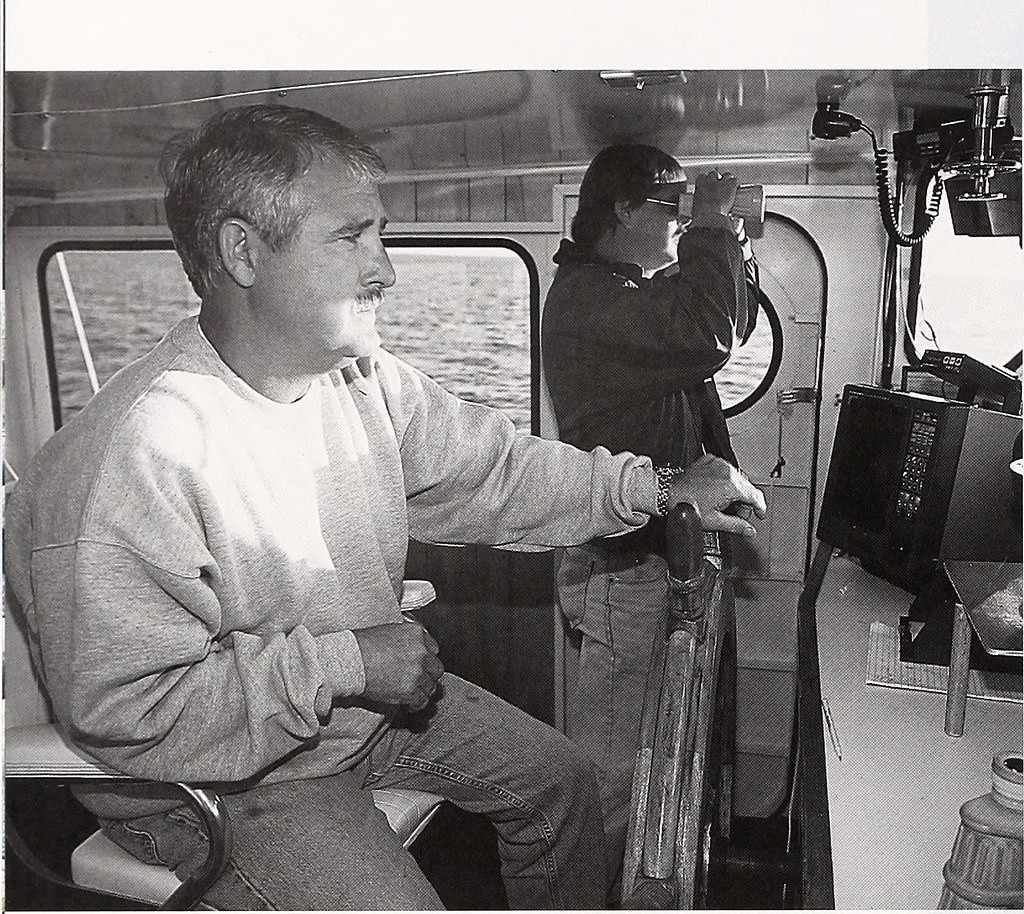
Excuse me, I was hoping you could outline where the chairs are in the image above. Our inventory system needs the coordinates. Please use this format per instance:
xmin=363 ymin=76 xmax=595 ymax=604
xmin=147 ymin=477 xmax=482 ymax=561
xmin=3 ymin=479 xmax=445 ymax=902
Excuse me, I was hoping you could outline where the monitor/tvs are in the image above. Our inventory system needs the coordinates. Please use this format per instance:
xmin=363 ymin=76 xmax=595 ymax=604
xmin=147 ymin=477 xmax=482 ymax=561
xmin=828 ymin=396 xmax=910 ymax=541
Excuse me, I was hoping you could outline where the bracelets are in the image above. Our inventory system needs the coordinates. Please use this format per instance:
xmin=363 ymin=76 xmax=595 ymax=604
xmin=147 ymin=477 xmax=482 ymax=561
xmin=656 ymin=467 xmax=673 ymax=516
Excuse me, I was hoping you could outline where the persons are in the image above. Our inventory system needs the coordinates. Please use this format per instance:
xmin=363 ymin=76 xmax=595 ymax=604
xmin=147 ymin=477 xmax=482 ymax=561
xmin=3 ymin=105 xmax=768 ymax=910
xmin=538 ymin=146 xmax=758 ymax=898
